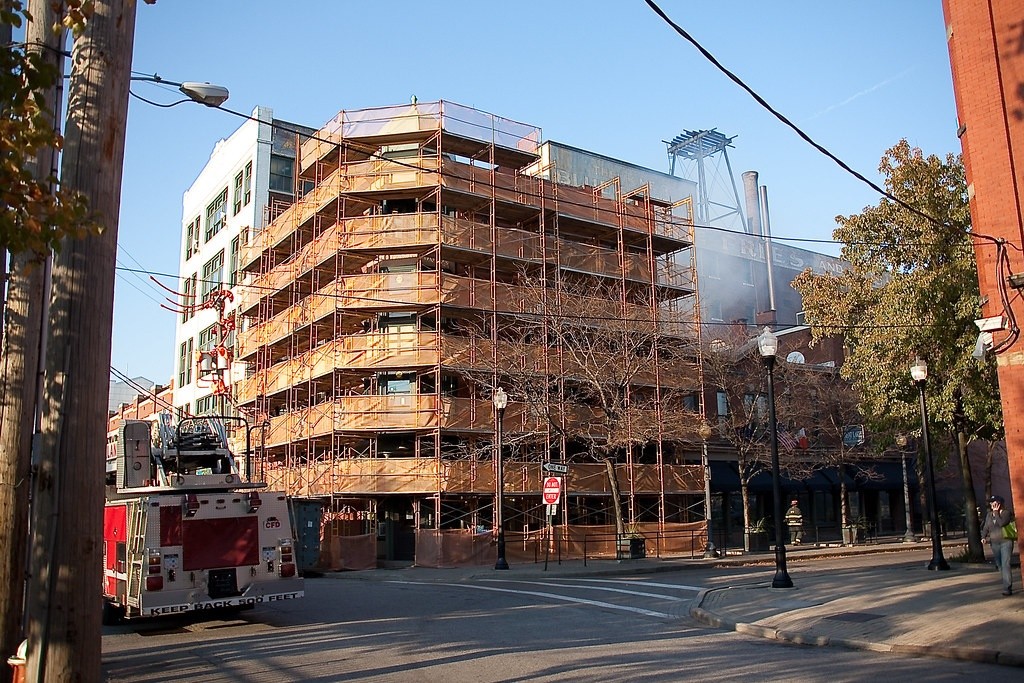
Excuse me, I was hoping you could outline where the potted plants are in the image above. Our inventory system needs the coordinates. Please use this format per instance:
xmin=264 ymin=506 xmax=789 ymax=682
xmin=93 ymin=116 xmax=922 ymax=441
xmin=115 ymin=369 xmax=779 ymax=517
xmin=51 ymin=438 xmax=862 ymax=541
xmin=844 ymin=513 xmax=874 ymax=545
xmin=750 ymin=517 xmax=769 ymax=552
xmin=618 ymin=518 xmax=645 ymax=559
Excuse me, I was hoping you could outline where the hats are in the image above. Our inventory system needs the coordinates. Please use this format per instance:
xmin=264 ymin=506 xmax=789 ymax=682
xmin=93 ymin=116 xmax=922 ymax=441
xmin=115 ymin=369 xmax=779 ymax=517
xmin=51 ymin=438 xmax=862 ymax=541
xmin=790 ymin=500 xmax=798 ymax=505
xmin=985 ymin=495 xmax=1005 ymax=504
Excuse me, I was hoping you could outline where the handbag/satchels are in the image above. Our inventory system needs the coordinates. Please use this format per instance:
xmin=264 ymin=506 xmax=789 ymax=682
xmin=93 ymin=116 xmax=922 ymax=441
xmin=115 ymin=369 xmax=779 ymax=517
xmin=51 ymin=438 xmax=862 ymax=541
xmin=1000 ymin=508 xmax=1017 ymax=539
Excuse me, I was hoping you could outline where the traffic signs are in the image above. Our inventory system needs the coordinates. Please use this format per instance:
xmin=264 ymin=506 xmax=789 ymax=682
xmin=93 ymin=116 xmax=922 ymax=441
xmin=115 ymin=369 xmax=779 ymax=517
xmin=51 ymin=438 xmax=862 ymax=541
xmin=540 ymin=460 xmax=568 ymax=475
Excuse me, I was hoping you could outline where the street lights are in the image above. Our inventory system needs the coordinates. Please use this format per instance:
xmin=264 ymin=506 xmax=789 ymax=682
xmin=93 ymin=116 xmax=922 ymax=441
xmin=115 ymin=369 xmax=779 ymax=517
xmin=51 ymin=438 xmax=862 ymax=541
xmin=492 ymin=386 xmax=509 ymax=572
xmin=755 ymin=326 xmax=795 ymax=589
xmin=893 ymin=431 xmax=917 ymax=543
xmin=906 ymin=354 xmax=951 ymax=571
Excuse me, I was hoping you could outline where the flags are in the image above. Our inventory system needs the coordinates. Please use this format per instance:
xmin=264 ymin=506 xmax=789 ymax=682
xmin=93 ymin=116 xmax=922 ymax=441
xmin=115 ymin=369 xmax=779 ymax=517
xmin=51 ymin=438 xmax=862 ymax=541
xmin=767 ymin=420 xmax=798 ymax=452
xmin=794 ymin=428 xmax=808 ymax=449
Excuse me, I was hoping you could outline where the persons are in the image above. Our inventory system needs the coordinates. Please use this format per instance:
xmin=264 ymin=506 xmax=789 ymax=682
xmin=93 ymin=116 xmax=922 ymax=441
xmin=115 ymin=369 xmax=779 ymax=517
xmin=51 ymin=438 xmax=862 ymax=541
xmin=785 ymin=500 xmax=803 ymax=546
xmin=981 ymin=495 xmax=1014 ymax=595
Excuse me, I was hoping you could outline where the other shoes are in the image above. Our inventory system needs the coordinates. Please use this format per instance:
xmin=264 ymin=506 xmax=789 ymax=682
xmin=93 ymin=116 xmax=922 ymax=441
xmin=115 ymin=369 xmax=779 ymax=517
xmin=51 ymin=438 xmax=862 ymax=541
xmin=1001 ymin=591 xmax=1013 ymax=596
xmin=791 ymin=544 xmax=797 ymax=546
xmin=795 ymin=541 xmax=803 ymax=545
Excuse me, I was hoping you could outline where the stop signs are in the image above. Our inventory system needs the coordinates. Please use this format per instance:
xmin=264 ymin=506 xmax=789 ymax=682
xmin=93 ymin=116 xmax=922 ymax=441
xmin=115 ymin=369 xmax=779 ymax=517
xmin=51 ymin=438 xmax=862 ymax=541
xmin=542 ymin=476 xmax=562 ymax=506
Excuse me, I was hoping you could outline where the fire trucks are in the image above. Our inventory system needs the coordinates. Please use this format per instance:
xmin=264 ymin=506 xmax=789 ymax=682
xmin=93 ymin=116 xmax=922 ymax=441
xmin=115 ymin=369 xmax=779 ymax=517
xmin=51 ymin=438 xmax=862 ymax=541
xmin=100 ymin=415 xmax=306 ymax=627
